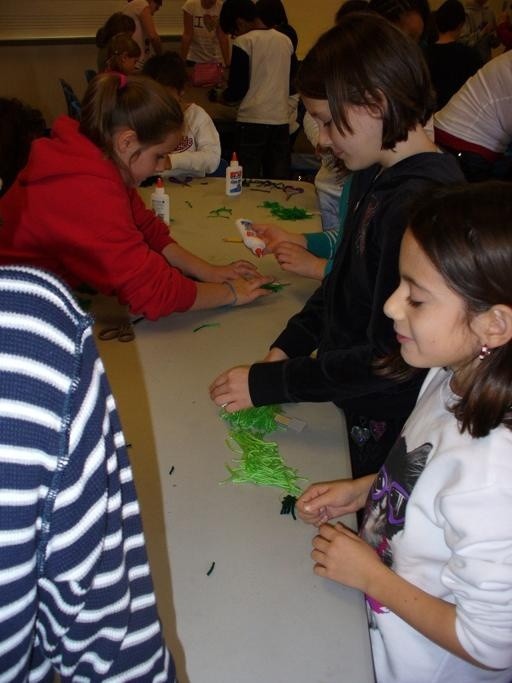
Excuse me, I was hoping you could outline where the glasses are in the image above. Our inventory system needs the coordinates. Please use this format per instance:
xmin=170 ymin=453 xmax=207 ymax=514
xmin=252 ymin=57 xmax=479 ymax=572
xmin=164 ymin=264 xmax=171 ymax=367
xmin=369 ymin=465 xmax=410 ymax=524
xmin=231 ymin=30 xmax=235 ymax=39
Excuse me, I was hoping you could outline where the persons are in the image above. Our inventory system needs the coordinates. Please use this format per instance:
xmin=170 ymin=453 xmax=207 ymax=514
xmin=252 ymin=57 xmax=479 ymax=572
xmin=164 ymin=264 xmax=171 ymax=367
xmin=296 ymin=195 xmax=511 ymax=677
xmin=120 ymin=0 xmax=159 ymax=51
xmin=252 ymin=170 xmax=352 ymax=281
xmin=181 ymin=1 xmax=232 ymax=78
xmin=98 ymin=36 xmax=141 ymax=67
xmin=216 ymin=2 xmax=291 ymax=173
xmin=423 ymin=4 xmax=486 ymax=104
xmin=133 ymin=56 xmax=222 ymax=182
xmin=259 ymin=4 xmax=301 ymax=169
xmin=424 ymin=47 xmax=509 ymax=179
xmin=303 ymin=1 xmax=424 ymax=230
xmin=0 ymin=268 xmax=176 ymax=683
xmin=0 ymin=70 xmax=278 ymax=319
xmin=209 ymin=20 xmax=473 ymax=480
xmin=94 ymin=13 xmax=134 ymax=67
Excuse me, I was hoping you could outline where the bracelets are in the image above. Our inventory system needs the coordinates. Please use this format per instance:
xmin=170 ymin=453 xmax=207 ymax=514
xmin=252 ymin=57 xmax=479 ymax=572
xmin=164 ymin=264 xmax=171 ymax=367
xmin=223 ymin=280 xmax=236 ymax=311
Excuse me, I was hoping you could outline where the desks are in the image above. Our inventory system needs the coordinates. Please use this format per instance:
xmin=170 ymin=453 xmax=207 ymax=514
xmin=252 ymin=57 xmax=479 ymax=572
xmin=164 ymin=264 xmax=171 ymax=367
xmin=87 ymin=176 xmax=376 ymax=682
xmin=160 ymin=84 xmax=322 ymax=153
